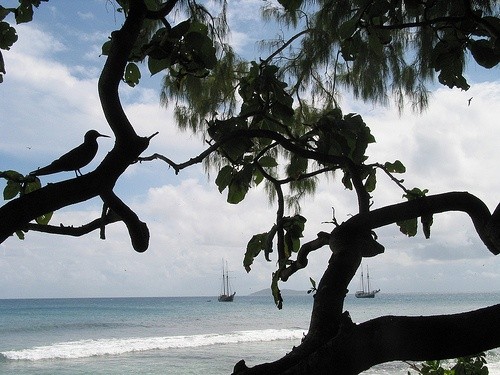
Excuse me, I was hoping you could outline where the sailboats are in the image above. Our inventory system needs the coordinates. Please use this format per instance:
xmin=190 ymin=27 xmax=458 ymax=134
xmin=354 ymin=263 xmax=381 ymax=299
xmin=218 ymin=261 xmax=237 ymax=303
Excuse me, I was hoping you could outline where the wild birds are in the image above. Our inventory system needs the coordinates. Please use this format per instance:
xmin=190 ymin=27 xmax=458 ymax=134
xmin=28 ymin=129 xmax=111 ymax=180
